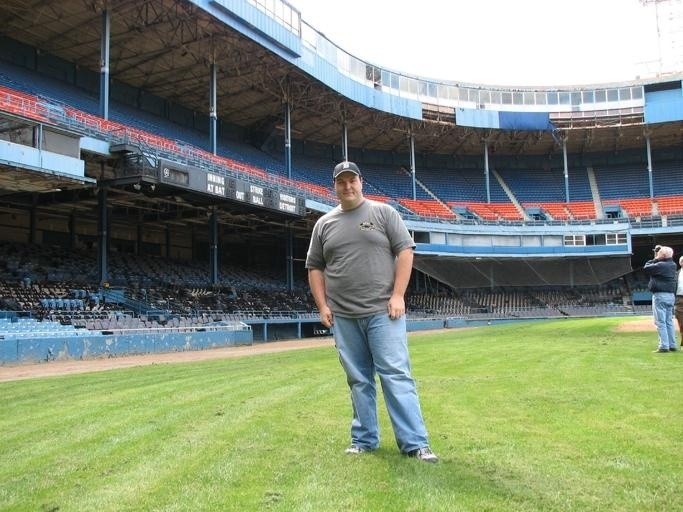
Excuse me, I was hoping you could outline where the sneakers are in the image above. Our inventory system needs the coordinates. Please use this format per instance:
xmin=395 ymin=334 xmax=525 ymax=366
xmin=345 ymin=445 xmax=366 ymax=454
xmin=653 ymin=348 xmax=668 ymax=353
xmin=408 ymin=446 xmax=439 ymax=462
xmin=668 ymin=348 xmax=677 ymax=351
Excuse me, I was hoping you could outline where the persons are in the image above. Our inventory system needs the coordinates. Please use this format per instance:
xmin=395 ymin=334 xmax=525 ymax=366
xmin=305 ymin=161 xmax=439 ymax=464
xmin=644 ymin=243 xmax=679 ymax=352
xmin=674 ymin=255 xmax=683 ymax=350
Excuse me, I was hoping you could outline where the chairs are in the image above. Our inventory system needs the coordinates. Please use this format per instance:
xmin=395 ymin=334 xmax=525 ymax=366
xmin=1 ymin=64 xmax=103 ymax=140
xmin=405 ymin=281 xmax=652 ymax=318
xmin=414 ymin=167 xmax=683 ymax=221
xmin=99 ymin=251 xmax=321 ymax=334
xmin=1 ymin=242 xmax=99 ymax=338
xmin=103 ymin=99 xmax=213 ymax=171
xmin=213 ymin=136 xmax=414 ymax=216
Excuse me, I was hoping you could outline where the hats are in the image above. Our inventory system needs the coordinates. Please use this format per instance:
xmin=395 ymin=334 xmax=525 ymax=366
xmin=332 ymin=161 xmax=361 ymax=179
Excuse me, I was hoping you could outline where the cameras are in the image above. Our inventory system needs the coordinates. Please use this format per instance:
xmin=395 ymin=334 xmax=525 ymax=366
xmin=652 ymin=247 xmax=661 ymax=258
